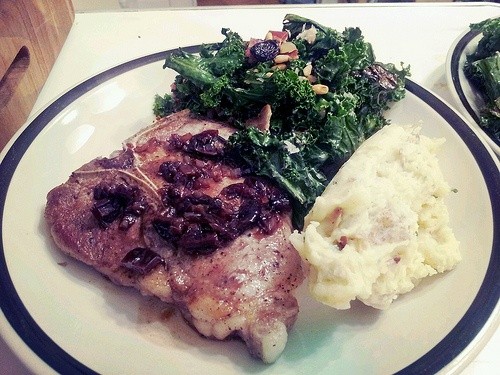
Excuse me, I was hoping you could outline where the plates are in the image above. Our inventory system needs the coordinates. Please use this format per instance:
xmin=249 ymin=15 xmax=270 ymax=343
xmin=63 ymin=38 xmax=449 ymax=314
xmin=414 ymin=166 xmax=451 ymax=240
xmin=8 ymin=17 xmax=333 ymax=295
xmin=448 ymin=15 xmax=500 ymax=153
xmin=0 ymin=43 xmax=499 ymax=375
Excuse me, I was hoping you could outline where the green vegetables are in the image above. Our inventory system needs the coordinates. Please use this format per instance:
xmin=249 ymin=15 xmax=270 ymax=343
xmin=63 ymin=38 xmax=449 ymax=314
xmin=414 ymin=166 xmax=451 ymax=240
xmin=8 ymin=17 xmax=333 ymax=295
xmin=465 ymin=16 xmax=500 ymax=143
xmin=153 ymin=14 xmax=412 ymax=234
